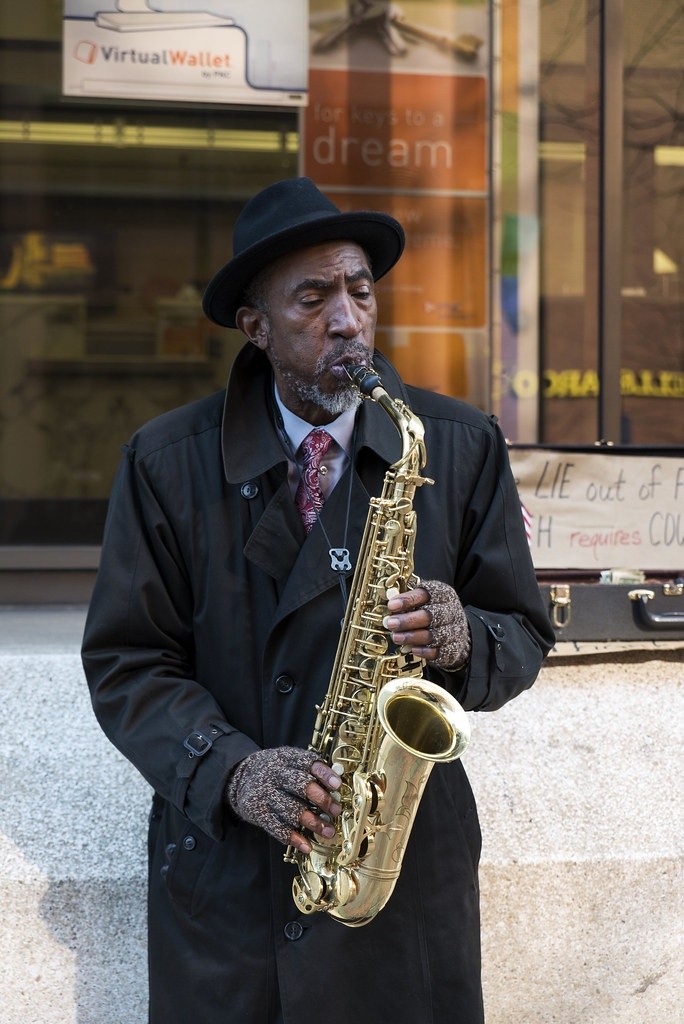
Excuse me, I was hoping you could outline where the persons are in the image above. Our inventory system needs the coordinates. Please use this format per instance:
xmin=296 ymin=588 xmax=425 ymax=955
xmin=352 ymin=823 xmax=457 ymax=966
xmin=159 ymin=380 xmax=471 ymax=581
xmin=80 ymin=175 xmax=557 ymax=1024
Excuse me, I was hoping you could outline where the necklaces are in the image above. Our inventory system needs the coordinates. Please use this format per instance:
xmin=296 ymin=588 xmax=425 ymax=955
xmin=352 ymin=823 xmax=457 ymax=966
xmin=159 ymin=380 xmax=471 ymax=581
xmin=271 ymin=395 xmax=360 ymax=612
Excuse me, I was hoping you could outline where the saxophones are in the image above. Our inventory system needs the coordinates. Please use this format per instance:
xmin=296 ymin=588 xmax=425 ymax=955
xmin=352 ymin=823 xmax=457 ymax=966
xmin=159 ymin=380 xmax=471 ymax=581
xmin=284 ymin=357 xmax=474 ymax=928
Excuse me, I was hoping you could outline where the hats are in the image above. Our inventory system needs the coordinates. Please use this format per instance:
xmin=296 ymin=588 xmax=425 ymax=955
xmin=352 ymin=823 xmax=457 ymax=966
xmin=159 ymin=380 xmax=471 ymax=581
xmin=202 ymin=177 xmax=407 ymax=330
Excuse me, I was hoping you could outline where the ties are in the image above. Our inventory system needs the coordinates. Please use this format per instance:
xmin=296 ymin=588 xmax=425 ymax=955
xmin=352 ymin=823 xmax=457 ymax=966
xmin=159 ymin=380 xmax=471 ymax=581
xmin=297 ymin=429 xmax=333 ymax=534
